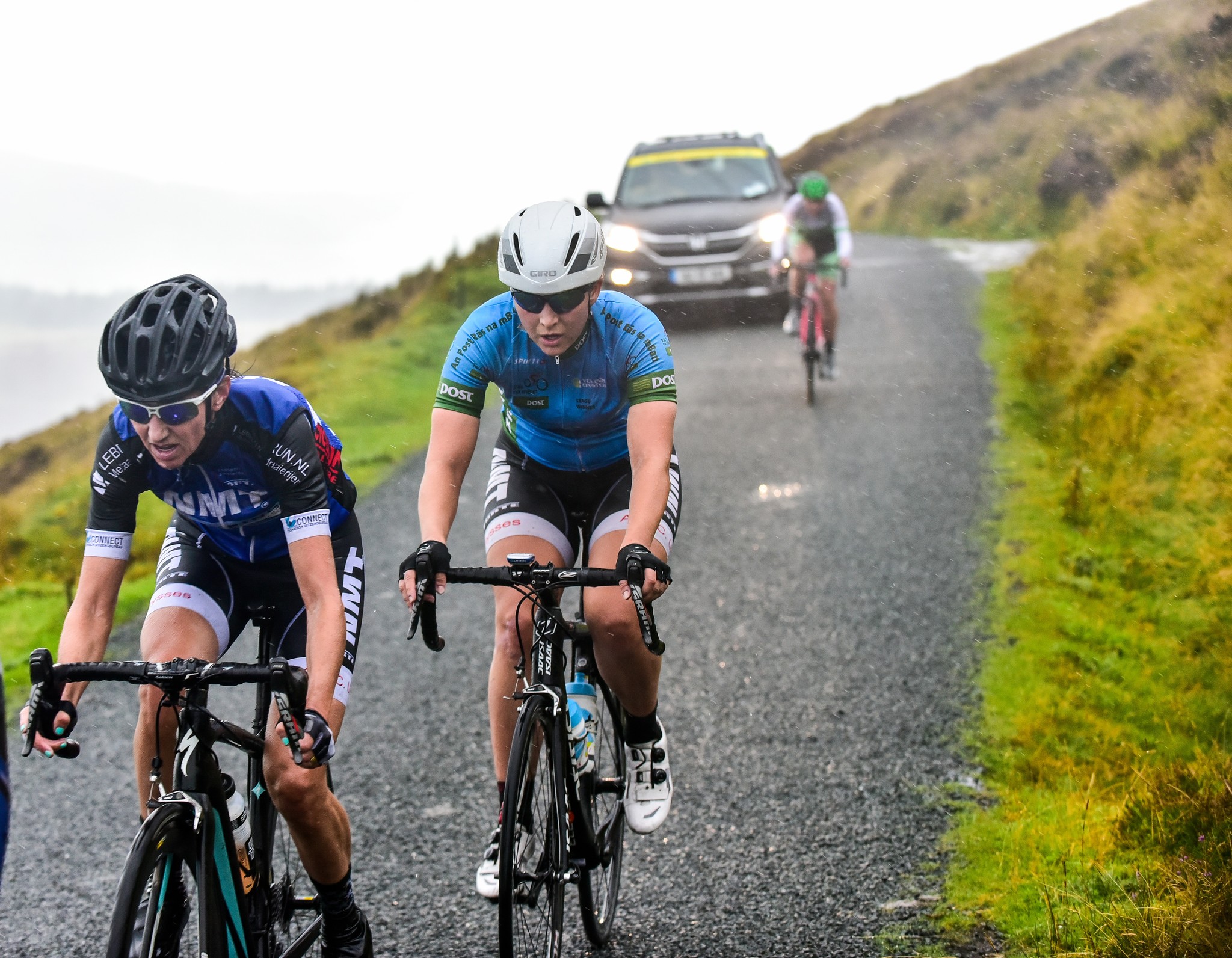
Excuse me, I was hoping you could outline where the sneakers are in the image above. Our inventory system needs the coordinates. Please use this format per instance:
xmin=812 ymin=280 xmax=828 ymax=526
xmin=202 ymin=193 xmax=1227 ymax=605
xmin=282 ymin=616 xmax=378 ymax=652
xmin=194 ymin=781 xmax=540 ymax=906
xmin=622 ymin=715 xmax=674 ymax=834
xmin=475 ymin=822 xmax=535 ymax=899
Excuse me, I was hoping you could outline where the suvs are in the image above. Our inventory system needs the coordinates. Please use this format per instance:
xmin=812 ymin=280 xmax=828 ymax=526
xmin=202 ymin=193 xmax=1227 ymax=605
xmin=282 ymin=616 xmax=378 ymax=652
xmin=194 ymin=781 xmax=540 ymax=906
xmin=583 ymin=130 xmax=800 ymax=322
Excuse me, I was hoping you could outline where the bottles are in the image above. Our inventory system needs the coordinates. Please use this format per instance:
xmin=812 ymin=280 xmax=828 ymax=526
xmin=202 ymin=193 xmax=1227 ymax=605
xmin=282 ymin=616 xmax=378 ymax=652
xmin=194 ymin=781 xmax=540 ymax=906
xmin=568 ymin=698 xmax=589 ymax=775
xmin=221 ymin=773 xmax=257 ymax=894
xmin=565 ymin=673 xmax=597 ymax=773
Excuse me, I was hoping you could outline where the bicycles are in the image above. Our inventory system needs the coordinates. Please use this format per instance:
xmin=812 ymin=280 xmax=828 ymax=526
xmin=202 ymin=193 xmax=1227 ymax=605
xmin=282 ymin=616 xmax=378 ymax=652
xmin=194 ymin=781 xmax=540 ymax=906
xmin=20 ymin=581 xmax=321 ymax=958
xmin=774 ymin=248 xmax=849 ymax=406
xmin=404 ymin=509 xmax=667 ymax=958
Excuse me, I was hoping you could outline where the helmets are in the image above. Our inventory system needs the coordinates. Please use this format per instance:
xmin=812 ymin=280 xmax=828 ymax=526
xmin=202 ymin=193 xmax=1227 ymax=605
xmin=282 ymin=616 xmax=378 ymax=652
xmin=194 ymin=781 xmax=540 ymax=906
xmin=798 ymin=175 xmax=828 ymax=201
xmin=98 ymin=274 xmax=238 ymax=406
xmin=498 ymin=201 xmax=607 ymax=296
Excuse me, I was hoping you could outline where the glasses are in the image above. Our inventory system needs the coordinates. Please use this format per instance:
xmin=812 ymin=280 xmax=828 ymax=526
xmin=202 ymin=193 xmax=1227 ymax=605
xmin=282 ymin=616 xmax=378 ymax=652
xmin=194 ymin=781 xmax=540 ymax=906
xmin=512 ymin=288 xmax=587 ymax=314
xmin=118 ymin=384 xmax=216 ymax=425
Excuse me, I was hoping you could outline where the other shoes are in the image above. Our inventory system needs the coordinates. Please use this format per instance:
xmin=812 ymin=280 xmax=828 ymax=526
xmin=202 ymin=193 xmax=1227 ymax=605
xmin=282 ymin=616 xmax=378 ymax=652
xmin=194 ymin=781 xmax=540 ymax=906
xmin=320 ymin=906 xmax=370 ymax=958
xmin=782 ymin=313 xmax=799 ymax=335
xmin=824 ymin=362 xmax=838 ymax=379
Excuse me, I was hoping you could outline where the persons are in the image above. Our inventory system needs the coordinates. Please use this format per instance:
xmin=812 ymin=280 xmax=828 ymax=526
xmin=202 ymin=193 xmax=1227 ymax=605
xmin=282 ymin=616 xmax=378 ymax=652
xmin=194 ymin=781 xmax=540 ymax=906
xmin=771 ymin=173 xmax=851 ymax=378
xmin=20 ymin=273 xmax=373 ymax=958
xmin=397 ymin=200 xmax=682 ymax=898
